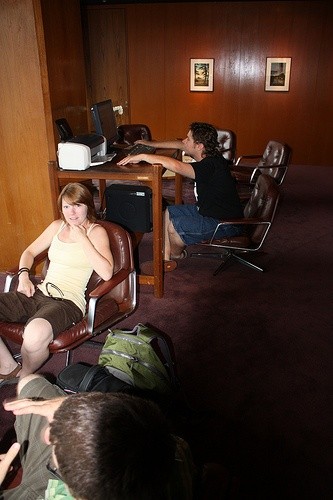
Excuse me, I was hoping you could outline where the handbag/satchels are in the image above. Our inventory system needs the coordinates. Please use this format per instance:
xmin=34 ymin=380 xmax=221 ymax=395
xmin=56 ymin=362 xmax=137 ymax=394
xmin=8 ymin=268 xmax=35 ymax=293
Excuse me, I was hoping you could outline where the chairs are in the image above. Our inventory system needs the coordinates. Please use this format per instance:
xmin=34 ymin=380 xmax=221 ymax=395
xmin=0 ymin=219 xmax=139 ymax=368
xmin=190 ymin=174 xmax=281 ymax=277
xmin=217 ymin=130 xmax=236 ymax=163
xmin=228 ymin=140 xmax=293 ymax=203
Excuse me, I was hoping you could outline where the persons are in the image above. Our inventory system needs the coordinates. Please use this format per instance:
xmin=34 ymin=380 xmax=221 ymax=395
xmin=116 ymin=122 xmax=244 ymax=274
xmin=0 ymin=183 xmax=113 ymax=388
xmin=0 ymin=374 xmax=193 ymax=500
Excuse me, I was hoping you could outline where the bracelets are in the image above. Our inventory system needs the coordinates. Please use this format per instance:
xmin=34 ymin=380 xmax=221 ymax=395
xmin=17 ymin=268 xmax=30 ymax=276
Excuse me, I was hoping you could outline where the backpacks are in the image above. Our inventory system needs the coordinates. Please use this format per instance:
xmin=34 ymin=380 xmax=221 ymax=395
xmin=98 ymin=323 xmax=171 ymax=391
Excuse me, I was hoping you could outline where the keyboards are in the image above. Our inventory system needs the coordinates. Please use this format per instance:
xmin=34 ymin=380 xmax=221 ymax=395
xmin=126 ymin=143 xmax=156 ymax=164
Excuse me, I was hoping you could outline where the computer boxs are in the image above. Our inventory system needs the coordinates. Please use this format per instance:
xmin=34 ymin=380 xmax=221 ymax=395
xmin=104 ymin=183 xmax=154 ymax=233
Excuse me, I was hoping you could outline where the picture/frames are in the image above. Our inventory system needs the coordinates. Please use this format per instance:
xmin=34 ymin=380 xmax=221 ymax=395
xmin=189 ymin=58 xmax=215 ymax=92
xmin=264 ymin=57 xmax=292 ymax=92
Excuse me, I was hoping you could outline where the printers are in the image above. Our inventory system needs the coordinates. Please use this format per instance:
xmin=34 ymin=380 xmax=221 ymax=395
xmin=55 ymin=118 xmax=117 ymax=171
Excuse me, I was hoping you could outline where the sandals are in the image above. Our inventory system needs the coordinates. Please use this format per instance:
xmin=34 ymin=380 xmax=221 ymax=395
xmin=0 ymin=362 xmax=22 ymax=387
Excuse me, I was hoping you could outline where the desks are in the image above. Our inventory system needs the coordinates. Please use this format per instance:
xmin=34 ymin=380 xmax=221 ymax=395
xmin=48 ymin=148 xmax=182 ymax=298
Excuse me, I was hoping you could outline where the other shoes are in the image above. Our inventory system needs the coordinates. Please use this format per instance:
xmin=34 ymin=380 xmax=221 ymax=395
xmin=139 ymin=249 xmax=187 ymax=274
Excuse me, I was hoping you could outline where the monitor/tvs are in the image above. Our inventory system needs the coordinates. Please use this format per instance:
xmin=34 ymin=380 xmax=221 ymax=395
xmin=92 ymin=99 xmax=119 ymax=154
